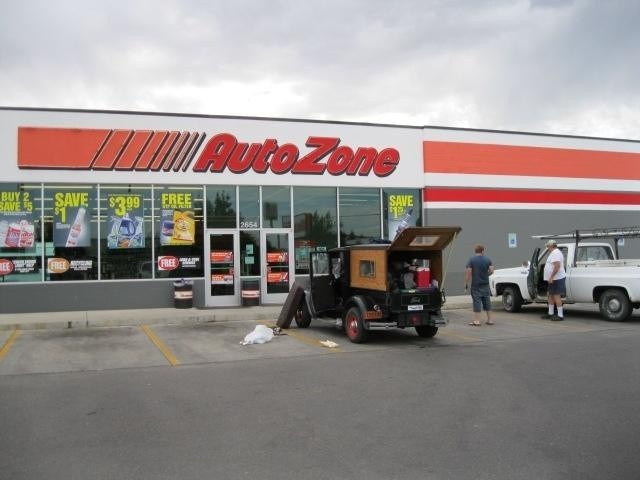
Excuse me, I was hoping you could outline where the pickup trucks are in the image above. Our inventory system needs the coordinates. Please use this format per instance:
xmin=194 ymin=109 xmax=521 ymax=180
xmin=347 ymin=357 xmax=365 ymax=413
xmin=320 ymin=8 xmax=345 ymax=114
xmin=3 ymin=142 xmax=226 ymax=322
xmin=486 ymin=228 xmax=640 ymax=323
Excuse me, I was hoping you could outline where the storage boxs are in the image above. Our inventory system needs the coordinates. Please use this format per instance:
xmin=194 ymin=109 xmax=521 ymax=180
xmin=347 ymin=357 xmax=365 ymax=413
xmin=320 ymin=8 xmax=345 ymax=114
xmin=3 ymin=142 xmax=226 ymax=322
xmin=407 ymin=265 xmax=431 ymax=288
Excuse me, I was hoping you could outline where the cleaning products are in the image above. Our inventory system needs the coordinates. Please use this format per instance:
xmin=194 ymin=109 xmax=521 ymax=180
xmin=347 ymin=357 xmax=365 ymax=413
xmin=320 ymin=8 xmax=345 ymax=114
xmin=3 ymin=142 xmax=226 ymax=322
xmin=128 ymin=216 xmax=144 ymax=248
xmin=106 ymin=216 xmax=122 ymax=248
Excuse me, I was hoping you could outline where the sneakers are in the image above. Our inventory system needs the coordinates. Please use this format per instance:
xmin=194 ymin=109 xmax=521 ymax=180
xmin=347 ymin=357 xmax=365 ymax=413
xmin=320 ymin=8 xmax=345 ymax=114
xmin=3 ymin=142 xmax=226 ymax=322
xmin=541 ymin=314 xmax=565 ymax=321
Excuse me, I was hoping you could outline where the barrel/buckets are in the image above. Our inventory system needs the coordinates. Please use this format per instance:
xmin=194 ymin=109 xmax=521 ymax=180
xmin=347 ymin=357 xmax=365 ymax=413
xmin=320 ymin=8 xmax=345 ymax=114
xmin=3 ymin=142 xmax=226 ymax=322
xmin=170 ymin=210 xmax=195 ymax=245
xmin=117 ymin=213 xmax=136 ymax=248
xmin=159 ymin=207 xmax=176 ymax=246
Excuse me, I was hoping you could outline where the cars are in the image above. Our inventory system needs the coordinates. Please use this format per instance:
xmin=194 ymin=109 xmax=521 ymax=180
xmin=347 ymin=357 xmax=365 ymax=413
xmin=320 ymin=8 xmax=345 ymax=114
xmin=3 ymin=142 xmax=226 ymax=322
xmin=140 ymin=260 xmax=168 ymax=279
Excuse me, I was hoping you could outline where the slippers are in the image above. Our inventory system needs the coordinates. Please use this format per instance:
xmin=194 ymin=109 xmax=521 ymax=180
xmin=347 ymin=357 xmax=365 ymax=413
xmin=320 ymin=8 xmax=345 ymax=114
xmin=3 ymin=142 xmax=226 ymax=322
xmin=468 ymin=321 xmax=480 ymax=326
xmin=485 ymin=320 xmax=494 ymax=325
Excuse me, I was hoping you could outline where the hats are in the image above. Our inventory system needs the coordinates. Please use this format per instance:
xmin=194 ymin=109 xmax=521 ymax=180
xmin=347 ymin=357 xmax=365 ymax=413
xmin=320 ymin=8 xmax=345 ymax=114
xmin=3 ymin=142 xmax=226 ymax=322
xmin=544 ymin=239 xmax=557 ymax=246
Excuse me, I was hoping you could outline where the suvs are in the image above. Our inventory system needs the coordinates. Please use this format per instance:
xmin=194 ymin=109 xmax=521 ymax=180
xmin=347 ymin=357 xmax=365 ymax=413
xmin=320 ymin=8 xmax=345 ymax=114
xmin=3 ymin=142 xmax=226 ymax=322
xmin=277 ymin=223 xmax=463 ymax=342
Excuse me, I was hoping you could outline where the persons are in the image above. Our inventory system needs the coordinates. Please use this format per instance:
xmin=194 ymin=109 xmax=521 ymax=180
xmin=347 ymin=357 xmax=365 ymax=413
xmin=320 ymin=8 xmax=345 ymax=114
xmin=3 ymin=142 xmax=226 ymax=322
xmin=463 ymin=244 xmax=495 ymax=327
xmin=539 ymin=238 xmax=567 ymax=322
